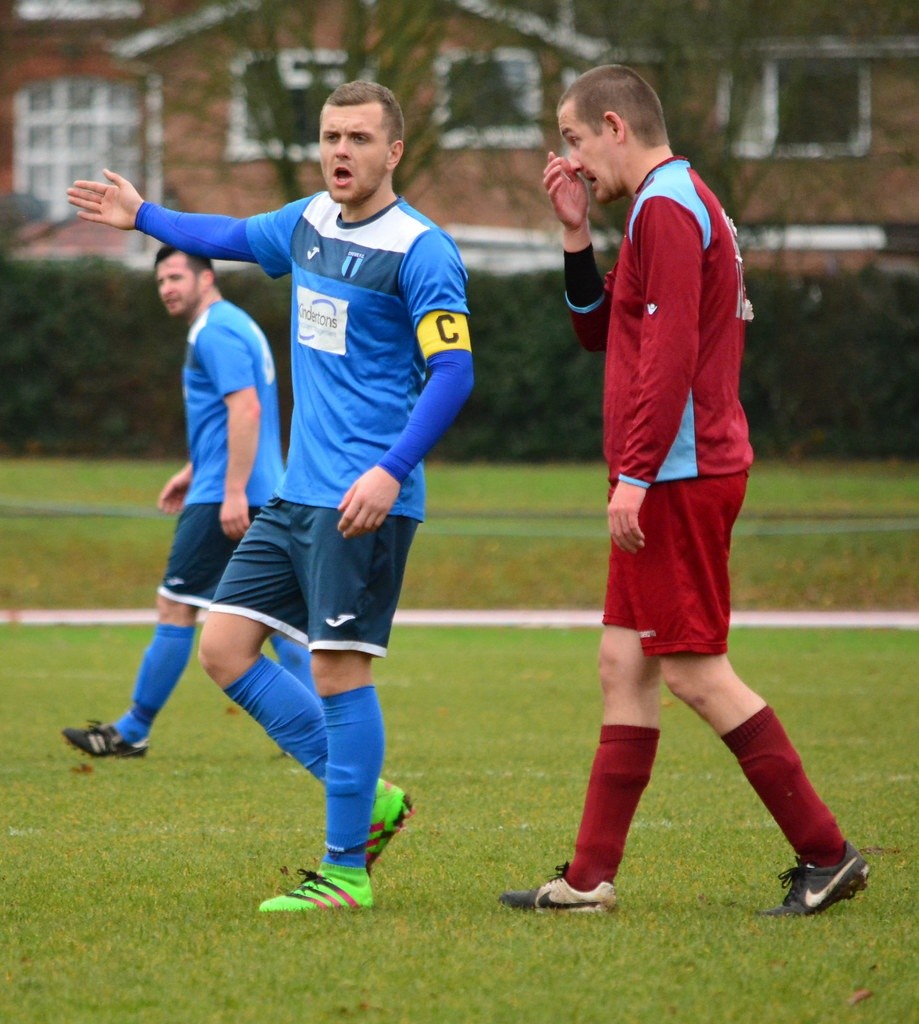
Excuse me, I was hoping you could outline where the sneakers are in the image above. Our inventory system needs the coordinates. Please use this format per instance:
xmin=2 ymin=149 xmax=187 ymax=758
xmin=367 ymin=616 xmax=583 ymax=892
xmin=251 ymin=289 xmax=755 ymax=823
xmin=364 ymin=777 xmax=417 ymax=870
xmin=754 ymin=841 xmax=871 ymax=916
xmin=60 ymin=719 xmax=148 ymax=757
xmin=260 ymin=861 xmax=373 ymax=911
xmin=497 ymin=862 xmax=615 ymax=912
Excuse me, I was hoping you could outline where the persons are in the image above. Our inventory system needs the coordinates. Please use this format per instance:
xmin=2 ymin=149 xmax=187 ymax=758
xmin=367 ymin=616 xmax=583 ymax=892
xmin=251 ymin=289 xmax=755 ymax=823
xmin=61 ymin=247 xmax=313 ymax=757
xmin=67 ymin=81 xmax=472 ymax=911
xmin=496 ymin=64 xmax=871 ymax=918
